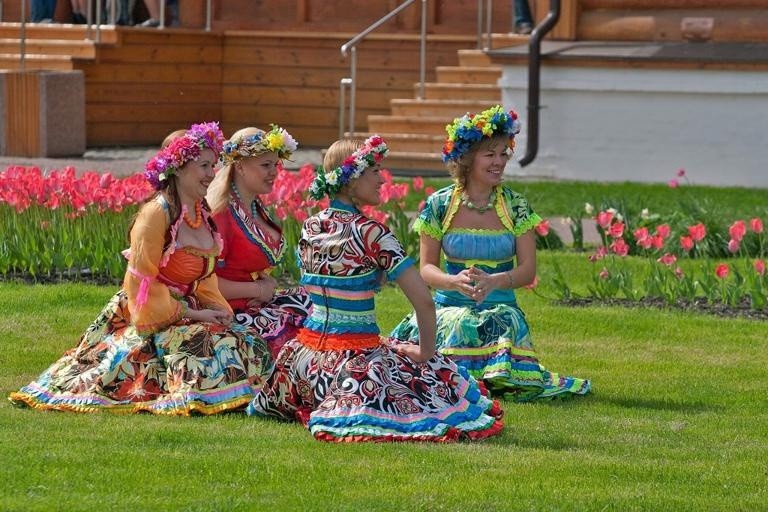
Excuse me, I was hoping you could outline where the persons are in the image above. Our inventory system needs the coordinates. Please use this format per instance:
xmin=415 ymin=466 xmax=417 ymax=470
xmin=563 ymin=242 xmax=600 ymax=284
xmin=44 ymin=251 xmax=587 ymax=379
xmin=30 ymin=0 xmax=180 ymax=27
xmin=513 ymin=0 xmax=536 ymax=34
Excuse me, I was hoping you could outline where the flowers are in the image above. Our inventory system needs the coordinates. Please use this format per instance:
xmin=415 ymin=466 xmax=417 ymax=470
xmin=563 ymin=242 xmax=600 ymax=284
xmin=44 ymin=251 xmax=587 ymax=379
xmin=144 ymin=118 xmax=224 ymax=191
xmin=442 ymin=104 xmax=521 ymax=163
xmin=308 ymin=134 xmax=389 ymax=202
xmin=223 ymin=123 xmax=299 ymax=163
